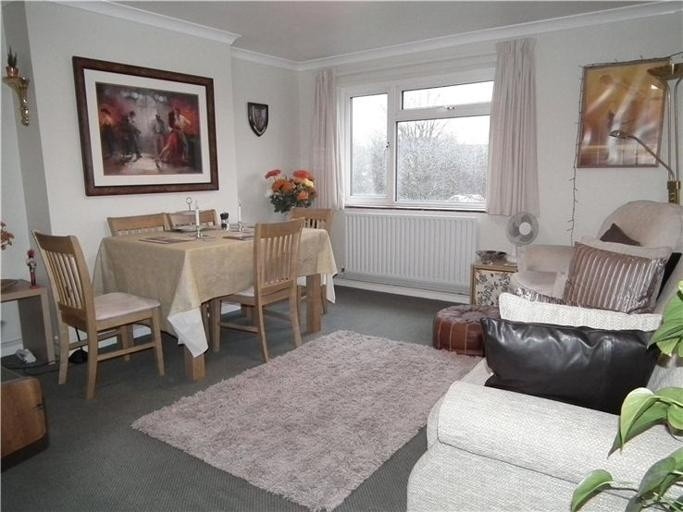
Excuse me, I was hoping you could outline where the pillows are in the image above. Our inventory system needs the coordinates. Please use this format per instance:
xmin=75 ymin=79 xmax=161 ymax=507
xmin=484 ymin=321 xmax=662 ymax=411
xmin=551 ymin=222 xmax=681 ymax=314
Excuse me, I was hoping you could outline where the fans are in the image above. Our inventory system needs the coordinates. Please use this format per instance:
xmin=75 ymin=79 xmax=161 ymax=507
xmin=509 ymin=211 xmax=540 ymax=264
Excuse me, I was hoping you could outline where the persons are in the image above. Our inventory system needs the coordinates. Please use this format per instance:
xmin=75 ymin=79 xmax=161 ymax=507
xmin=253 ymin=107 xmax=264 ymax=130
xmin=99 ymin=105 xmax=193 ymax=169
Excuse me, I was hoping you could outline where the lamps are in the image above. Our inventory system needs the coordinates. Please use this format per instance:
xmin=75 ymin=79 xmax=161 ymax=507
xmin=609 ymin=63 xmax=683 ymax=206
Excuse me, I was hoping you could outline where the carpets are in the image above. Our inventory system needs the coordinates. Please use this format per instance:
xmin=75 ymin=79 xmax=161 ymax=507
xmin=133 ymin=329 xmax=483 ymax=510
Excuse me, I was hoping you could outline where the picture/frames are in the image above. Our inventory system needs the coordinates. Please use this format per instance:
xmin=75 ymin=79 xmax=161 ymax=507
xmin=248 ymin=101 xmax=271 ymax=137
xmin=68 ymin=56 xmax=220 ymax=195
xmin=575 ymin=56 xmax=668 ymax=169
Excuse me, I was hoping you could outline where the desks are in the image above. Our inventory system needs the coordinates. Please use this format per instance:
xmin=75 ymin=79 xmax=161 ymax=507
xmin=1 ymin=277 xmax=56 ymax=369
xmin=470 ymin=262 xmax=518 ymax=309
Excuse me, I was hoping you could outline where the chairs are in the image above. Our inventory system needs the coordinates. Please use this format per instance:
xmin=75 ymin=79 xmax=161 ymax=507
xmin=405 ymin=291 xmax=683 ymax=511
xmin=511 ymin=199 xmax=683 ymax=310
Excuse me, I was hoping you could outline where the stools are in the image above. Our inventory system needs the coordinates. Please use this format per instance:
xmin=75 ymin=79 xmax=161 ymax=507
xmin=431 ymin=304 xmax=500 ymax=357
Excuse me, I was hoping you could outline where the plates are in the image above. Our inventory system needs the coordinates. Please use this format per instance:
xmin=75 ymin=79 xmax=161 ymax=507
xmin=172 ymin=222 xmax=256 ymax=240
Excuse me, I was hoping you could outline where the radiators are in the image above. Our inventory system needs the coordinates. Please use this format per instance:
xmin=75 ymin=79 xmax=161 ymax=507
xmin=344 ymin=211 xmax=478 ymax=299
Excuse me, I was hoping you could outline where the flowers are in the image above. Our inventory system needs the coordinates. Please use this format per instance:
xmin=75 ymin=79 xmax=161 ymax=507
xmin=265 ymin=169 xmax=314 ymax=213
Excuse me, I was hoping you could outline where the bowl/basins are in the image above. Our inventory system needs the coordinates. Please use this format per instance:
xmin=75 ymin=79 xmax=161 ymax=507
xmin=475 ymin=249 xmax=508 ymax=266
